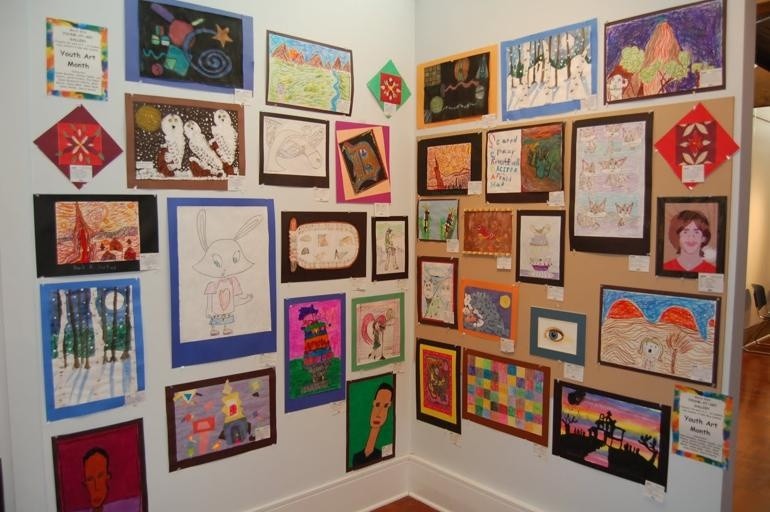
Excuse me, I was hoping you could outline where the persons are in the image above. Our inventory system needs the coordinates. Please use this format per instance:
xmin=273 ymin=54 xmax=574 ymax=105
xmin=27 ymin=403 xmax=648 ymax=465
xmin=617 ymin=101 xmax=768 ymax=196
xmin=662 ymin=208 xmax=718 ymax=273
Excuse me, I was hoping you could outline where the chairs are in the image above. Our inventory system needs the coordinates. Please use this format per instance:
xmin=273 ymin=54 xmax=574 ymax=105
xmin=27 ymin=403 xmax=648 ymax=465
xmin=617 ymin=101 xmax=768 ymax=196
xmin=743 ymin=284 xmax=770 ymax=356
